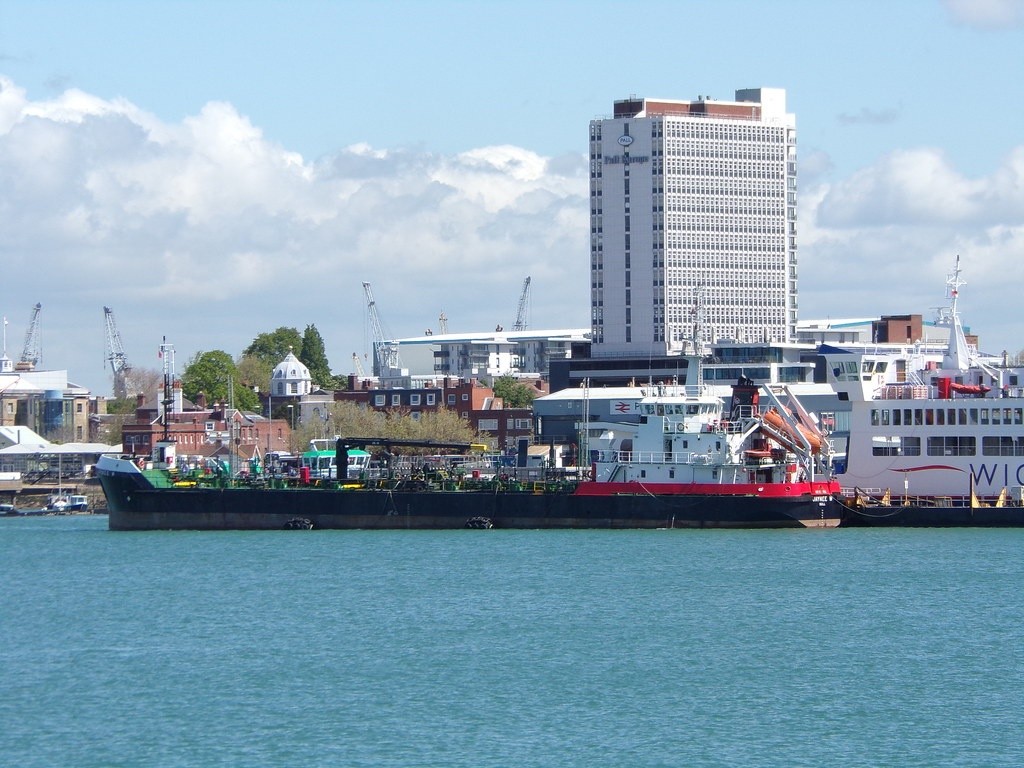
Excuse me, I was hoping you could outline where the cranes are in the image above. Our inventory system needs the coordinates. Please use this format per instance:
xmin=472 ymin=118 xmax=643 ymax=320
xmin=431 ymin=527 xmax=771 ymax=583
xmin=352 ymin=353 xmax=366 ymax=377
xmin=439 ymin=309 xmax=447 ymax=335
xmin=362 ymin=281 xmax=385 ymax=362
xmin=102 ymin=305 xmax=126 ymax=373
xmin=513 ymin=276 xmax=531 ymax=331
xmin=21 ymin=302 xmax=43 ymax=365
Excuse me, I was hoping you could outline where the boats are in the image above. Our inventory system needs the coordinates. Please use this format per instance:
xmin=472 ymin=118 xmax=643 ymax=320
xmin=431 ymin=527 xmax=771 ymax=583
xmin=96 ymin=285 xmax=848 ymax=531
xmin=45 ymin=494 xmax=88 ymax=512
xmin=815 ymin=254 xmax=1024 ymax=528
xmin=0 ymin=505 xmax=13 ymax=511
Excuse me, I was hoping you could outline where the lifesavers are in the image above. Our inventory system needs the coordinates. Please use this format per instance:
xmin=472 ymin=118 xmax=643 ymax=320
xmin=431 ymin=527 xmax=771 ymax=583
xmin=677 ymin=422 xmax=685 ymax=432
